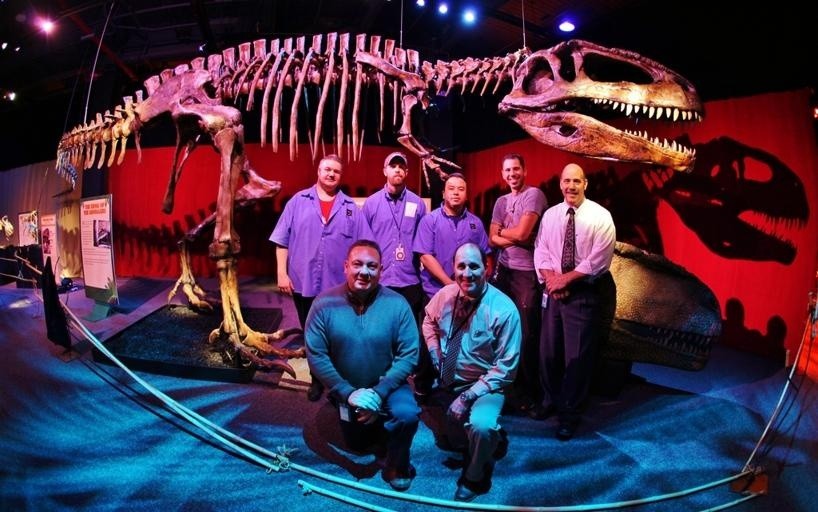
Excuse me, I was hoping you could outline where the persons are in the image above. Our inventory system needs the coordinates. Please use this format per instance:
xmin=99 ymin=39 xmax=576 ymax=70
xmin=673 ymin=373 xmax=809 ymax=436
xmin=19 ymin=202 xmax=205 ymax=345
xmin=413 ymin=174 xmax=492 ymax=406
xmin=364 ymin=151 xmax=426 ymax=308
xmin=269 ymin=154 xmax=362 ymax=397
xmin=419 ymin=243 xmax=522 ymax=502
xmin=304 ymin=241 xmax=423 ymax=491
xmin=488 ymin=152 xmax=549 ymax=411
xmin=528 ymin=163 xmax=616 ymax=426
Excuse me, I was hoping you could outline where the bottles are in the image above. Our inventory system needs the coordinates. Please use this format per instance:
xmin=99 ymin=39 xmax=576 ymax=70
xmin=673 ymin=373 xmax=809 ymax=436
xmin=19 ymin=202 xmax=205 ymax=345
xmin=384 ymin=152 xmax=407 ymax=167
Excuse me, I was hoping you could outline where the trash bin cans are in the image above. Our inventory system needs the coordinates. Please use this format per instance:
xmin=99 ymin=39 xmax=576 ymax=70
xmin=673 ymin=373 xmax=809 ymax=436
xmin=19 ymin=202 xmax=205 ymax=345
xmin=460 ymin=392 xmax=470 ymax=403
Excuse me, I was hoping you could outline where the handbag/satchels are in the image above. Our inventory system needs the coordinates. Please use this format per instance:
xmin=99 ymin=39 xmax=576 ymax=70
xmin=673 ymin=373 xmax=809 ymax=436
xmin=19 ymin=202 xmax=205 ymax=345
xmin=497 ymin=227 xmax=503 ymax=237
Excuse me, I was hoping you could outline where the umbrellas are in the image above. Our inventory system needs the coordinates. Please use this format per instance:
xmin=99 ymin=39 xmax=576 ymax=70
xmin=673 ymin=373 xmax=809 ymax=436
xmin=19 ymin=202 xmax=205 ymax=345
xmin=454 ymin=484 xmax=476 ymax=502
xmin=390 ymin=477 xmax=411 ymax=491
xmin=308 ymin=383 xmax=325 ymax=401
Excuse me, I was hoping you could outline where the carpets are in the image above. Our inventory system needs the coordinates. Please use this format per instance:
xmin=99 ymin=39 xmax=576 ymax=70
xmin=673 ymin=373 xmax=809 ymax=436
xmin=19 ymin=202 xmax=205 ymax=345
xmin=440 ymin=297 xmax=469 ymax=389
xmin=560 ymin=208 xmax=575 ymax=274
